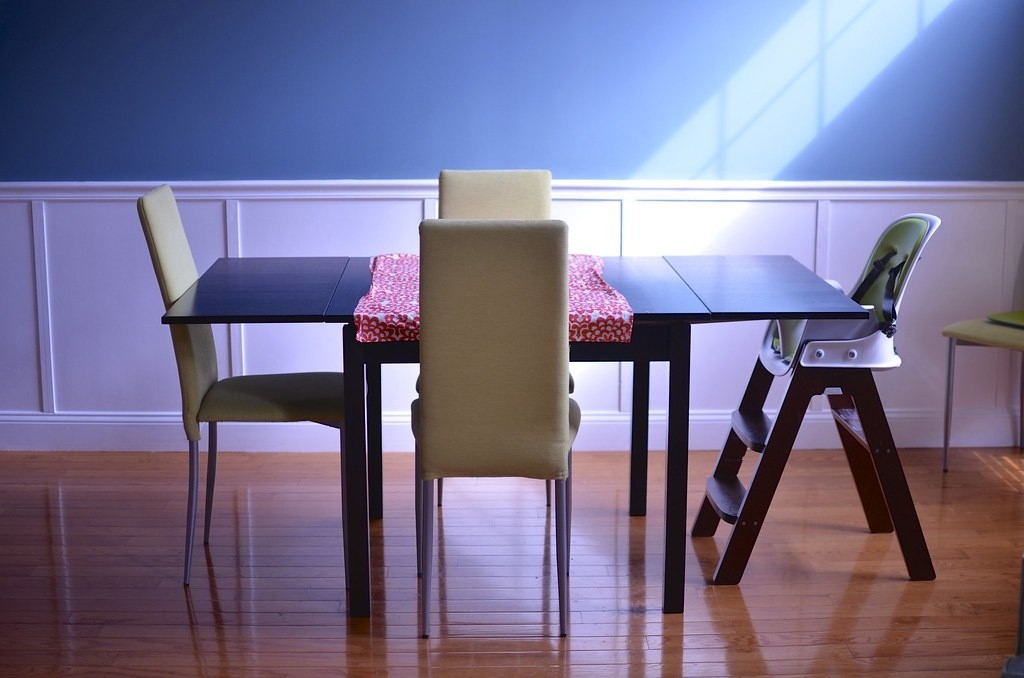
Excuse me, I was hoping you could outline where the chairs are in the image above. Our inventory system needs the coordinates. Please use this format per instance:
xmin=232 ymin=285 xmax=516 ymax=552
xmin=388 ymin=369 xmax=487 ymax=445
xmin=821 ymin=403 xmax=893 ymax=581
xmin=693 ymin=212 xmax=942 ymax=585
xmin=415 ymin=168 xmax=574 ymax=506
xmin=410 ymin=220 xmax=581 ymax=641
xmin=135 ymin=183 xmax=346 ymax=585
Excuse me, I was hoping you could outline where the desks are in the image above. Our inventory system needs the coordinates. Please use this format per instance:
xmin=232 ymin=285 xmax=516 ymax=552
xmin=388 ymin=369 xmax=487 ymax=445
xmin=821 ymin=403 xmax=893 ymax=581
xmin=162 ymin=251 xmax=870 ymax=616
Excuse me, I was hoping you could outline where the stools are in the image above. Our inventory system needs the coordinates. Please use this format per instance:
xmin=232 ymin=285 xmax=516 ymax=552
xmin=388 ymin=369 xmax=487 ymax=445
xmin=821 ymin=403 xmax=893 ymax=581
xmin=942 ymin=311 xmax=1024 ymax=472
